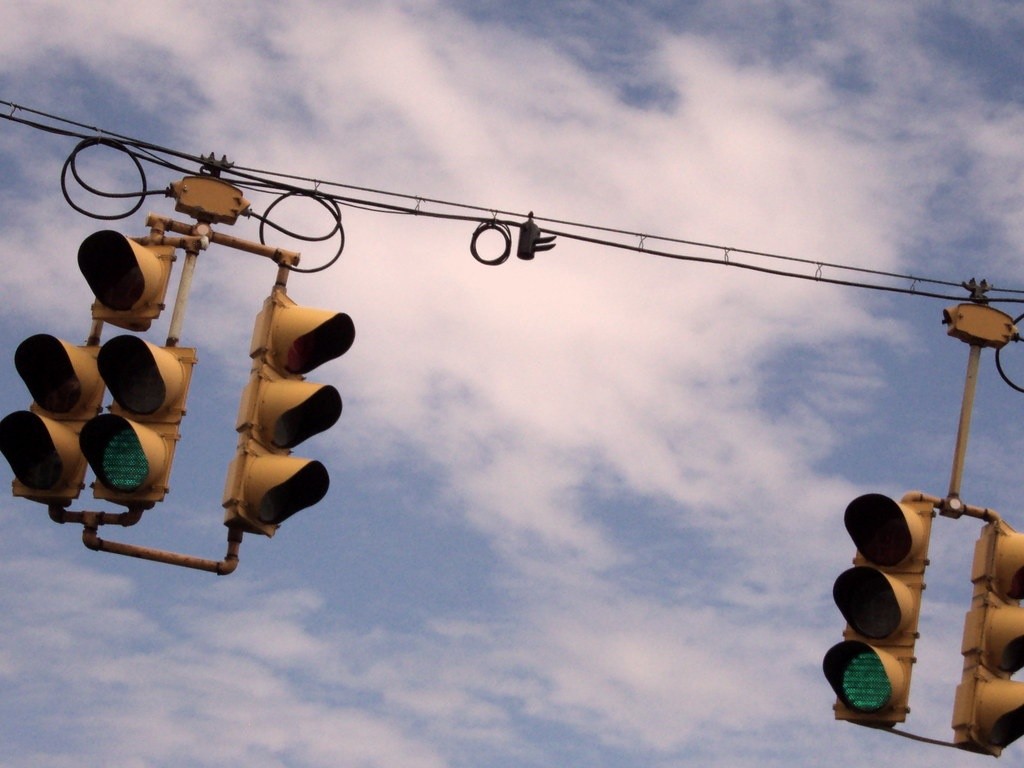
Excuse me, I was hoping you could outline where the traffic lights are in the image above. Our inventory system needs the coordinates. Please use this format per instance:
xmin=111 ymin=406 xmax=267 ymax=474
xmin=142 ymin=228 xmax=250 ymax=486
xmin=0 ymin=330 xmax=111 ymax=503
xmin=951 ymin=524 xmax=1024 ymax=759
xmin=75 ymin=228 xmax=178 ymax=333
xmin=226 ymin=291 xmax=358 ymax=544
xmin=77 ymin=334 xmax=194 ymax=505
xmin=822 ymin=490 xmax=935 ymax=725
